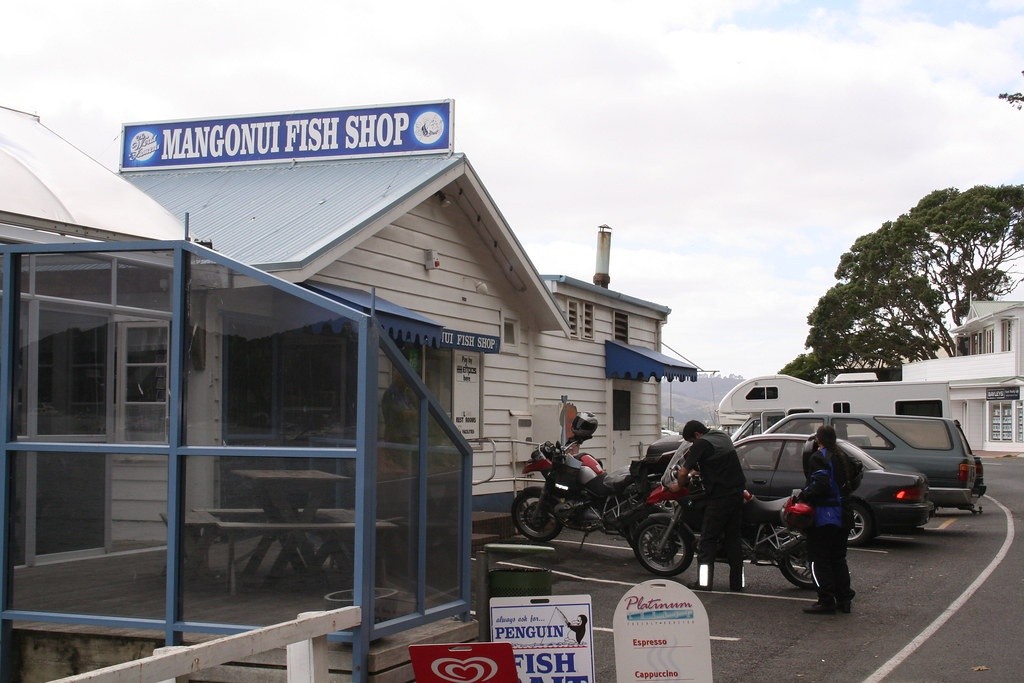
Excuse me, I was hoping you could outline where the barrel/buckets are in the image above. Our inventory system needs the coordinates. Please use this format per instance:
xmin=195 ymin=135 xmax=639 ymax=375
xmin=324 ymin=587 xmax=399 ymax=646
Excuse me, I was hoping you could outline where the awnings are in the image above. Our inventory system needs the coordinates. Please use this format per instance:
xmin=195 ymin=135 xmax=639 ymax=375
xmin=303 ymin=278 xmax=445 ymax=349
xmin=605 ymin=340 xmax=697 ymax=382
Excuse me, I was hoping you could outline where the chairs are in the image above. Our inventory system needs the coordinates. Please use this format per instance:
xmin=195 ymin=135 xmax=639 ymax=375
xmin=772 ymin=448 xmax=792 ymax=473
xmin=793 ymin=423 xmax=813 ymax=436
xmin=834 ymin=423 xmax=849 ymax=441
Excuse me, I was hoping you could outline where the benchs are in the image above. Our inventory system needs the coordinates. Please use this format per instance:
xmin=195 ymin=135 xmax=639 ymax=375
xmin=159 ymin=507 xmax=402 ymax=592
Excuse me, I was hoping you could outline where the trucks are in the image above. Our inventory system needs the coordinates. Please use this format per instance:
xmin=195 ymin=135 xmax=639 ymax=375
xmin=716 ymin=375 xmax=955 ymax=442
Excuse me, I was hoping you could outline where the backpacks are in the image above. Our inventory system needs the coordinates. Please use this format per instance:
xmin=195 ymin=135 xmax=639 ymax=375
xmin=831 ymin=451 xmax=864 ymax=493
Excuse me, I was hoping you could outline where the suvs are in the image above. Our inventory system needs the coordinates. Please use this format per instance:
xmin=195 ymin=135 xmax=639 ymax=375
xmin=764 ymin=413 xmax=988 ymax=517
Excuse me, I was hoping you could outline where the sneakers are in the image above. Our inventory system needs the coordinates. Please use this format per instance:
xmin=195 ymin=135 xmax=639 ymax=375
xmin=802 ymin=602 xmax=852 ymax=614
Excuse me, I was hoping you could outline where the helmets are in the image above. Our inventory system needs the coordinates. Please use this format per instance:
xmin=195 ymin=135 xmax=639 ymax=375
xmin=780 ymin=494 xmax=814 ymax=530
xmin=571 ymin=412 xmax=599 ymax=437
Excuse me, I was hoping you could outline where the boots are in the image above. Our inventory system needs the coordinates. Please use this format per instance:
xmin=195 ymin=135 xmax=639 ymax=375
xmin=730 ymin=565 xmax=745 ymax=591
xmin=687 ymin=562 xmax=713 ymax=590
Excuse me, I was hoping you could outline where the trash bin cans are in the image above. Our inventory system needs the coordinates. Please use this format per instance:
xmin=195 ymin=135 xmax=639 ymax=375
xmin=475 ymin=543 xmax=553 ymax=642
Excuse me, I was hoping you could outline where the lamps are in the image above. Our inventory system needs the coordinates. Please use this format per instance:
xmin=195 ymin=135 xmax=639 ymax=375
xmin=462 ymin=277 xmax=487 ymax=295
xmin=436 ymin=191 xmax=451 ymax=208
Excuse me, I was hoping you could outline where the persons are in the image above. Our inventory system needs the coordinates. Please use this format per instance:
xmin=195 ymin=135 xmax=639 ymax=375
xmin=794 ymin=426 xmax=853 ymax=615
xmin=677 ymin=419 xmax=748 ymax=593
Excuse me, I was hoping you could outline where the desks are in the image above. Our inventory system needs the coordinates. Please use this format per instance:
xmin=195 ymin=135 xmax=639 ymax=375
xmin=232 ymin=470 xmax=355 ymax=594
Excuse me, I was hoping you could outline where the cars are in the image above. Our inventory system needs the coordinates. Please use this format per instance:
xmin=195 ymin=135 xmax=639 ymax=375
xmin=731 ymin=434 xmax=930 ymax=546
xmin=661 ymin=430 xmax=679 ymax=437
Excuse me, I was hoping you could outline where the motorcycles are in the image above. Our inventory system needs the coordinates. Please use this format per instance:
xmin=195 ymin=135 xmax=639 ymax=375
xmin=510 ymin=424 xmax=680 ymax=549
xmin=632 ymin=450 xmax=819 ymax=588
